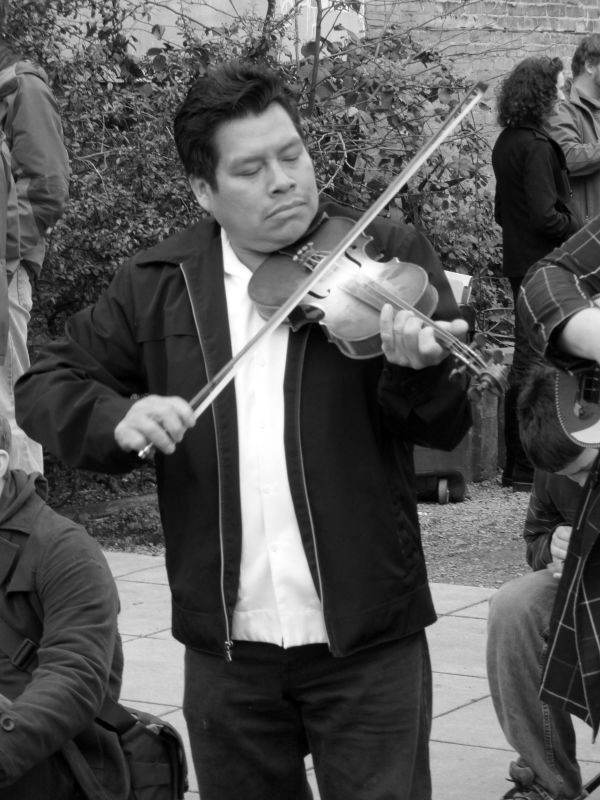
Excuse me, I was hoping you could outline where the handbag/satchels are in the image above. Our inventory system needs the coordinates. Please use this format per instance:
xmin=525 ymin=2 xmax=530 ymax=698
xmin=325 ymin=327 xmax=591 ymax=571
xmin=117 ymin=706 xmax=190 ymax=800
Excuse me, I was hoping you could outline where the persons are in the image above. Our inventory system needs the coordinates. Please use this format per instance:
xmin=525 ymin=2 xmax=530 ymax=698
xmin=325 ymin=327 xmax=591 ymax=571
xmin=11 ymin=63 xmax=480 ymax=800
xmin=0 ymin=415 xmax=135 ymax=800
xmin=0 ymin=36 xmax=74 ymax=478
xmin=491 ymin=36 xmax=600 ymax=800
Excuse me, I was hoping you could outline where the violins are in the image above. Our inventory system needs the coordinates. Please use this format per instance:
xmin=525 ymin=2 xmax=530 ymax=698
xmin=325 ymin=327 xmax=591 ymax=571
xmin=245 ymin=214 xmax=511 ymax=406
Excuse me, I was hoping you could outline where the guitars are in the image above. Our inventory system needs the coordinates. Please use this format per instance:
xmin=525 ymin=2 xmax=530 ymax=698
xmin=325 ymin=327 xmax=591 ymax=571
xmin=555 ymin=293 xmax=600 ymax=450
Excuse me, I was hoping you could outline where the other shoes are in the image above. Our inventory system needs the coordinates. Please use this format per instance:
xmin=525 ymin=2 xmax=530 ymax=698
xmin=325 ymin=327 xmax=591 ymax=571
xmin=508 ymin=784 xmax=587 ymax=800
xmin=501 ymin=465 xmax=535 ymax=492
